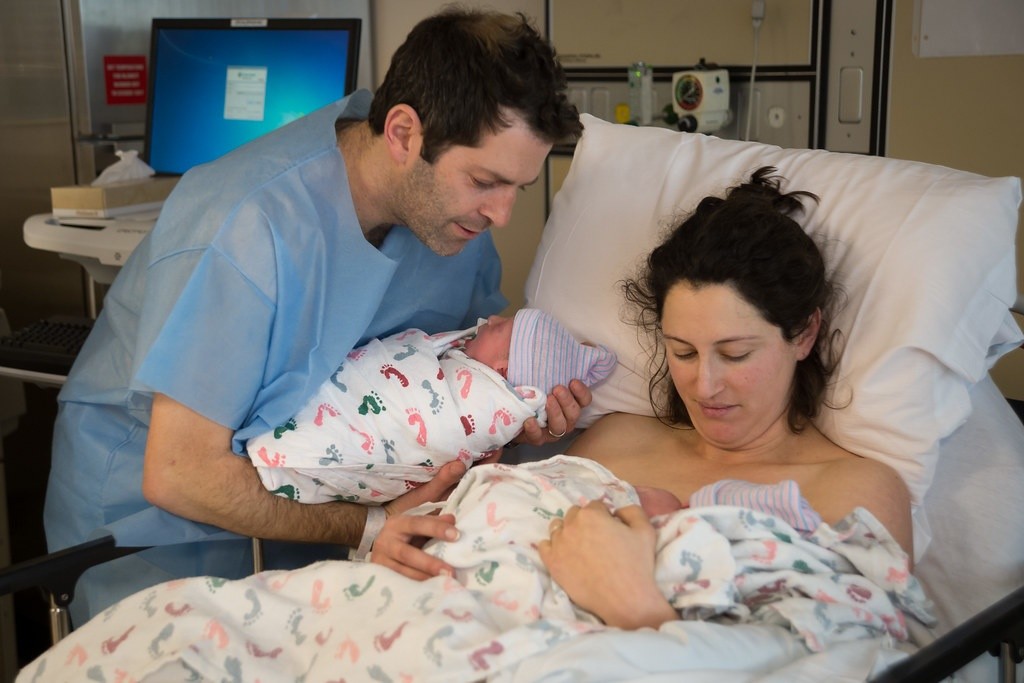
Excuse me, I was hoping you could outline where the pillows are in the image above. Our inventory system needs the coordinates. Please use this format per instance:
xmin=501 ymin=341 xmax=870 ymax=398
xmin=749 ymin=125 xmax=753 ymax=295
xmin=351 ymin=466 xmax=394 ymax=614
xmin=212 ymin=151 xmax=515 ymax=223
xmin=521 ymin=111 xmax=1024 ymax=566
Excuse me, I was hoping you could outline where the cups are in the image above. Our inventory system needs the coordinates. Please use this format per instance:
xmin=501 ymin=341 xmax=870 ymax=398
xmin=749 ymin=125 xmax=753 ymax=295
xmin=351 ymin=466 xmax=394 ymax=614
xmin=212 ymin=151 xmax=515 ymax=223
xmin=593 ymin=87 xmax=609 ymax=120
xmin=569 ymin=87 xmax=587 ymax=113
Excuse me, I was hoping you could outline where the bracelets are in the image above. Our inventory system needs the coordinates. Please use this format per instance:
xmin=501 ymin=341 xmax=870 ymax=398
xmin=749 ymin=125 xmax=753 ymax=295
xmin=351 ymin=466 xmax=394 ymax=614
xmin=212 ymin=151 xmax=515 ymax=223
xmin=349 ymin=506 xmax=386 ymax=560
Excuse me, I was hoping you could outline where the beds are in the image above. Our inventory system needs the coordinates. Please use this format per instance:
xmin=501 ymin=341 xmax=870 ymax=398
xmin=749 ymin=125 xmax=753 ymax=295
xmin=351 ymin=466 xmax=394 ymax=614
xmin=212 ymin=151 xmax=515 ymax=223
xmin=0 ymin=372 xmax=1024 ymax=683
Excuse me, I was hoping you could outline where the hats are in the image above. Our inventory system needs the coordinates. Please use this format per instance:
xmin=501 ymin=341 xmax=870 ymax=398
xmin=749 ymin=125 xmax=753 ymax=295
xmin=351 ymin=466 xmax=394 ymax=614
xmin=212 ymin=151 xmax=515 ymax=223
xmin=687 ymin=479 xmax=822 ymax=532
xmin=506 ymin=307 xmax=617 ymax=388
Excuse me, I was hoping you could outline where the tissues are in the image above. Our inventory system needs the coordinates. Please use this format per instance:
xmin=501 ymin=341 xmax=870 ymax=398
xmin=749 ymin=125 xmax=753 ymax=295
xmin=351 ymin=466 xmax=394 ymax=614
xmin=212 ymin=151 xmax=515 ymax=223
xmin=50 ymin=147 xmax=181 ymax=220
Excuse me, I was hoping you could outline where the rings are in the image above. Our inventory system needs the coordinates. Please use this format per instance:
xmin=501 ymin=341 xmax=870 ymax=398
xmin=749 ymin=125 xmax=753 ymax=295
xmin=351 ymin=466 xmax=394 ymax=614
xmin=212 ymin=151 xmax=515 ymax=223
xmin=547 ymin=521 xmax=562 ymax=535
xmin=548 ymin=428 xmax=567 ymax=439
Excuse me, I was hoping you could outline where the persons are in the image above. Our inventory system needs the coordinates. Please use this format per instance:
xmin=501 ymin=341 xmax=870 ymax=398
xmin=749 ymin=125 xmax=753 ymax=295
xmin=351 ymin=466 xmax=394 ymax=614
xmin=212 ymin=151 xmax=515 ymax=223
xmin=536 ymin=167 xmax=914 ymax=632
xmin=43 ymin=8 xmax=618 ymax=632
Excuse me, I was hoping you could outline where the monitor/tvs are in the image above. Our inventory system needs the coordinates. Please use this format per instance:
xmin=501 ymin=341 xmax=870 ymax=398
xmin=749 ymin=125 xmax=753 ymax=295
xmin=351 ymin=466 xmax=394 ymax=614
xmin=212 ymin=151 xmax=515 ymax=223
xmin=140 ymin=17 xmax=361 ymax=178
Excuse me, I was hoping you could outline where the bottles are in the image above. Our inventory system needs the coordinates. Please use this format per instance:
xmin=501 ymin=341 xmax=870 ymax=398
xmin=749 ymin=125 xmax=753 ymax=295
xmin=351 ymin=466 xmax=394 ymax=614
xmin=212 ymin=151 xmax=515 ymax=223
xmin=630 ymin=63 xmax=651 ymax=126
xmin=614 ymin=105 xmax=631 ymax=126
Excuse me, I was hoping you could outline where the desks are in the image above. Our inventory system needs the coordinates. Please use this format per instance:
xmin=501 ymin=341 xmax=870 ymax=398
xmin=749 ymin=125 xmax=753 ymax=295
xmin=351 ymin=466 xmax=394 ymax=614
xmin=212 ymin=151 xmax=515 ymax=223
xmin=21 ymin=212 xmax=159 ymax=319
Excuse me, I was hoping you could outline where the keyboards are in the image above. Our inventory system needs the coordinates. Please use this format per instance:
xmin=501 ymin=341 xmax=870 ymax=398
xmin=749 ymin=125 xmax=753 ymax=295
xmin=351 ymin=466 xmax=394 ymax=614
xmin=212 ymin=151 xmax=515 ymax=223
xmin=0 ymin=315 xmax=92 ymax=375
